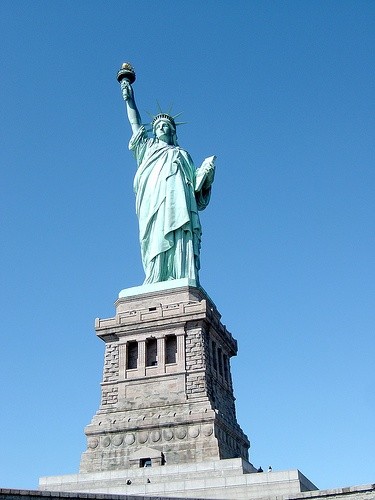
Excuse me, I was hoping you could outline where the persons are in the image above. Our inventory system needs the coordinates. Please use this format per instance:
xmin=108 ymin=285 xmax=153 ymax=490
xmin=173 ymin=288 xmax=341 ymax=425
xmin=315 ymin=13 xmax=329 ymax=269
xmin=268 ymin=465 xmax=273 ymax=473
xmin=121 ymin=81 xmax=216 ymax=285
xmin=257 ymin=465 xmax=264 ymax=473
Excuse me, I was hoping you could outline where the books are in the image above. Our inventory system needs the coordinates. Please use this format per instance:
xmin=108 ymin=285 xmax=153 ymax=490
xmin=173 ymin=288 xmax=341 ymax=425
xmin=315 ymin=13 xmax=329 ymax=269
xmin=194 ymin=155 xmax=216 ymax=193
